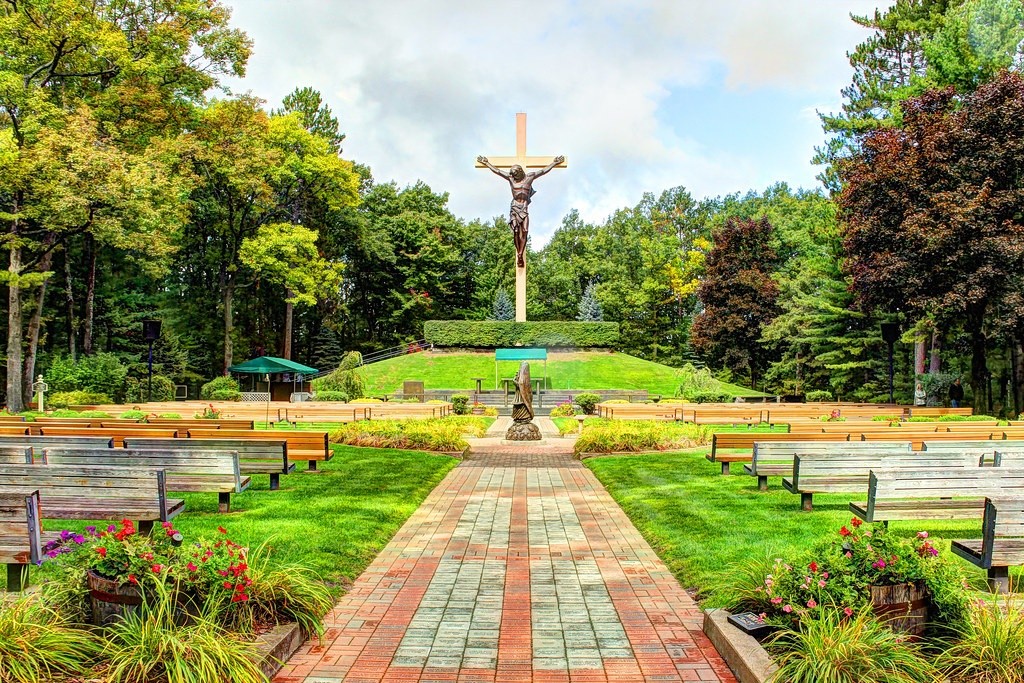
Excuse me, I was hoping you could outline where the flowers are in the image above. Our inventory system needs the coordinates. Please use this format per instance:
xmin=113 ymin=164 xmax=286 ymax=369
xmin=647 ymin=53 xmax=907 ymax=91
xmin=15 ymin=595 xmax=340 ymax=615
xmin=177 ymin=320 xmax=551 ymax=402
xmin=767 ymin=530 xmax=977 ymax=620
xmin=52 ymin=523 xmax=261 ymax=604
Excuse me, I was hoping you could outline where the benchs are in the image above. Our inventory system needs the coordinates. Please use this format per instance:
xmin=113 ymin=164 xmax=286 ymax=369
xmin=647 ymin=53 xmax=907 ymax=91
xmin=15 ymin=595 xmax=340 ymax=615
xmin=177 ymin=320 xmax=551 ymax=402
xmin=591 ymin=401 xmax=1024 ymax=591
xmin=0 ymin=401 xmax=454 ymax=578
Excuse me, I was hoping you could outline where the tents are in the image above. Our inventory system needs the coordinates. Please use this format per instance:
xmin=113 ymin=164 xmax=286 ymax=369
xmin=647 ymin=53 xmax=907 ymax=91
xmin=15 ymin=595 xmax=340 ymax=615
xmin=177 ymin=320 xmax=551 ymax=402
xmin=228 ymin=356 xmax=318 ymax=402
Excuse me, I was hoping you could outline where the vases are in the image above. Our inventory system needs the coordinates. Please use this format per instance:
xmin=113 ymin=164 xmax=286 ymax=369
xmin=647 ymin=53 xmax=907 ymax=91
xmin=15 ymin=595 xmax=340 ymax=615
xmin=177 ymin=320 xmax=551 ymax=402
xmin=88 ymin=570 xmax=183 ymax=638
xmin=836 ymin=579 xmax=948 ymax=645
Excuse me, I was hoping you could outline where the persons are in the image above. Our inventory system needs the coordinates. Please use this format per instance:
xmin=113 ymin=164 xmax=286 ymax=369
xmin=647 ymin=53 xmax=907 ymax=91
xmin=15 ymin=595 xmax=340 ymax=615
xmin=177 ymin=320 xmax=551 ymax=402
xmin=296 ymin=373 xmax=303 ymax=382
xmin=948 ymin=378 xmax=964 ymax=408
xmin=915 ymin=383 xmax=926 ymax=407
xmin=513 ymin=360 xmax=534 ymax=419
xmin=478 ymin=155 xmax=565 ymax=267
xmin=283 ymin=374 xmax=291 ymax=382
